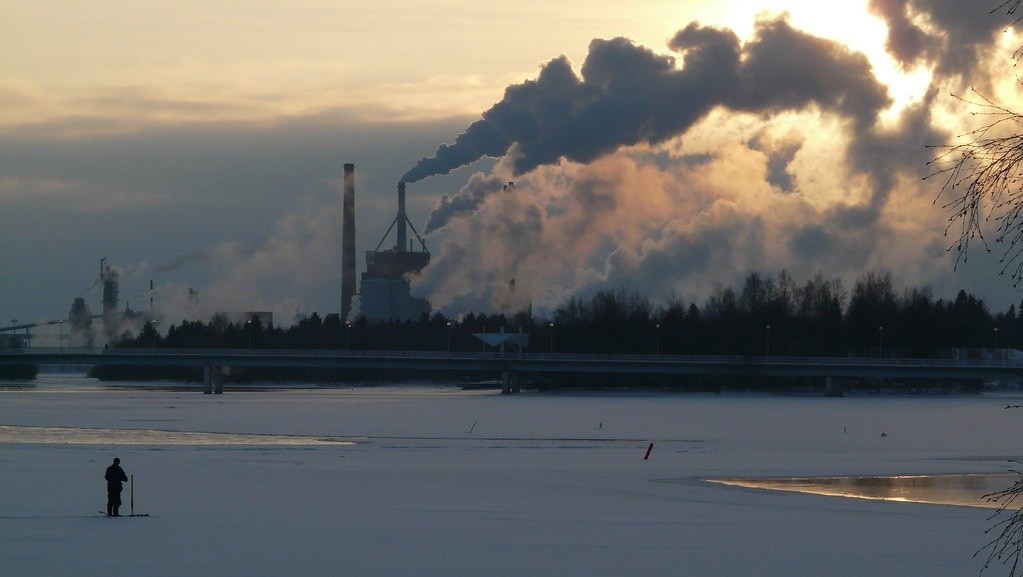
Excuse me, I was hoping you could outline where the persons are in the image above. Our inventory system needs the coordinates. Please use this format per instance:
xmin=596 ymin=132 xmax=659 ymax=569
xmin=105 ymin=342 xmax=111 ymax=351
xmin=105 ymin=458 xmax=128 ymax=517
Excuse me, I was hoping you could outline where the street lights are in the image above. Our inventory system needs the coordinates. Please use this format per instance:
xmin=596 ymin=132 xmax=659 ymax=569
xmin=247 ymin=320 xmax=251 ymax=353
xmin=11 ymin=319 xmax=18 ymax=337
xmin=446 ymin=321 xmax=451 ymax=357
xmin=153 ymin=319 xmax=156 ymax=353
xmin=878 ymin=326 xmax=884 ymax=364
xmin=58 ymin=319 xmax=64 ymax=355
xmin=766 ymin=325 xmax=770 ymax=360
xmin=345 ymin=320 xmax=351 ymax=353
xmin=550 ymin=323 xmax=553 ymax=356
xmin=656 ymin=324 xmax=661 ymax=360
xmin=993 ymin=328 xmax=999 ymax=360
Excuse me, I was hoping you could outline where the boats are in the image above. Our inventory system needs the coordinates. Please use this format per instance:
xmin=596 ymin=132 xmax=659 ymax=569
xmin=454 ymin=378 xmax=515 ymax=391
